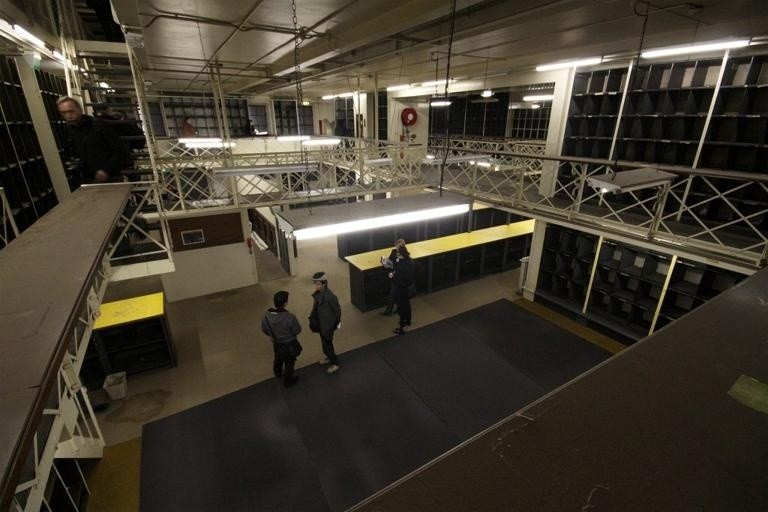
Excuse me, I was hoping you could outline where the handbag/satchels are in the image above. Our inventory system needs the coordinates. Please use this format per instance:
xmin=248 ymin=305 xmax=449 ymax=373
xmin=309 ymin=309 xmax=321 ymax=333
xmin=273 ymin=344 xmax=298 ymax=363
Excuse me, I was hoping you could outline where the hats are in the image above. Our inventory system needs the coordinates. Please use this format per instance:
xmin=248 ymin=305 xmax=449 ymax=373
xmin=312 ymin=272 xmax=327 ymax=282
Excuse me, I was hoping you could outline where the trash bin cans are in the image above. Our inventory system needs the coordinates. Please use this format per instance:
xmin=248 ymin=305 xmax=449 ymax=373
xmin=103 ymin=371 xmax=128 ymax=400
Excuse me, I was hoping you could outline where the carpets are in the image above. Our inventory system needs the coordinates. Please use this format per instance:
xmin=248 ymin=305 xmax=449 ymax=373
xmin=372 ymin=296 xmax=616 ymax=442
xmin=142 ymin=343 xmax=462 ymax=512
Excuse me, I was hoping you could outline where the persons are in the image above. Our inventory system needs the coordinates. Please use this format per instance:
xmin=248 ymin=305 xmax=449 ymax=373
xmin=379 ymin=238 xmax=405 ymax=316
xmin=183 ymin=116 xmax=199 ymax=156
xmin=52 ymin=95 xmax=147 ymax=256
xmin=261 ymin=291 xmax=302 ymax=387
xmin=392 ymin=246 xmax=415 ymax=336
xmin=246 ymin=120 xmax=257 ymax=137
xmin=310 ymin=272 xmax=342 ymax=375
xmin=94 ymin=101 xmax=147 ymax=208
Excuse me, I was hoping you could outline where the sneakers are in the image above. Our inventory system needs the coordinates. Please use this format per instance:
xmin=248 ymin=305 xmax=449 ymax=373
xmin=320 ymin=359 xmax=329 ymax=365
xmin=393 ymin=328 xmax=405 ymax=335
xmin=284 ymin=377 xmax=299 ymax=389
xmin=326 ymin=365 xmax=338 ymax=374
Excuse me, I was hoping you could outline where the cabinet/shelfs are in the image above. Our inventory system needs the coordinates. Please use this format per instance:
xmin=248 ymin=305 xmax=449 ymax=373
xmin=94 ymin=290 xmax=180 ymax=374
xmin=145 ymin=89 xmax=316 ymax=140
xmin=514 ymin=104 xmax=552 ymax=140
xmin=536 ymin=220 xmax=748 ymax=340
xmin=249 ymin=204 xmax=294 ymax=274
xmin=0 ymin=56 xmax=81 ymax=252
xmin=556 ymin=48 xmax=767 ymax=234
xmin=334 ymin=86 xmax=389 ymax=140
xmin=331 ymin=207 xmax=533 ymax=315
